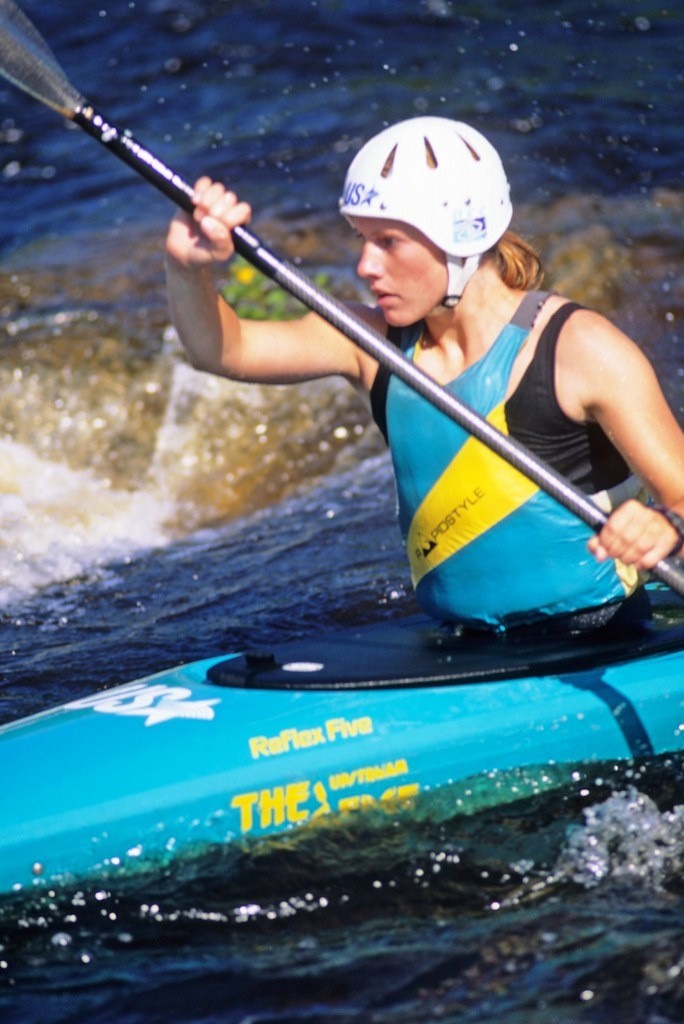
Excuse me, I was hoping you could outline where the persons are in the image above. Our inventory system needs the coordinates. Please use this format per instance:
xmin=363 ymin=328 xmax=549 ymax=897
xmin=162 ymin=113 xmax=684 ymax=652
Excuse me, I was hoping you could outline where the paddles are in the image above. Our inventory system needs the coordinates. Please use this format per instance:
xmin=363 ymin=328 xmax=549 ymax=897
xmin=0 ymin=0 xmax=682 ymax=599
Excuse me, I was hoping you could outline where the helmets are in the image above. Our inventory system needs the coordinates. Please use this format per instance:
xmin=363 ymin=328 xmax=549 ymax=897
xmin=346 ymin=116 xmax=513 ymax=311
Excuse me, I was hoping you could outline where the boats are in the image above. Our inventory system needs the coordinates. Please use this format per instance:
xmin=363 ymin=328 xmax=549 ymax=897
xmin=1 ymin=570 xmax=684 ymax=911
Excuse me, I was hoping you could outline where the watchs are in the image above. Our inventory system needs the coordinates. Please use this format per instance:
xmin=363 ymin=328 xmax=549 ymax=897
xmin=654 ymin=506 xmax=684 ymax=556
